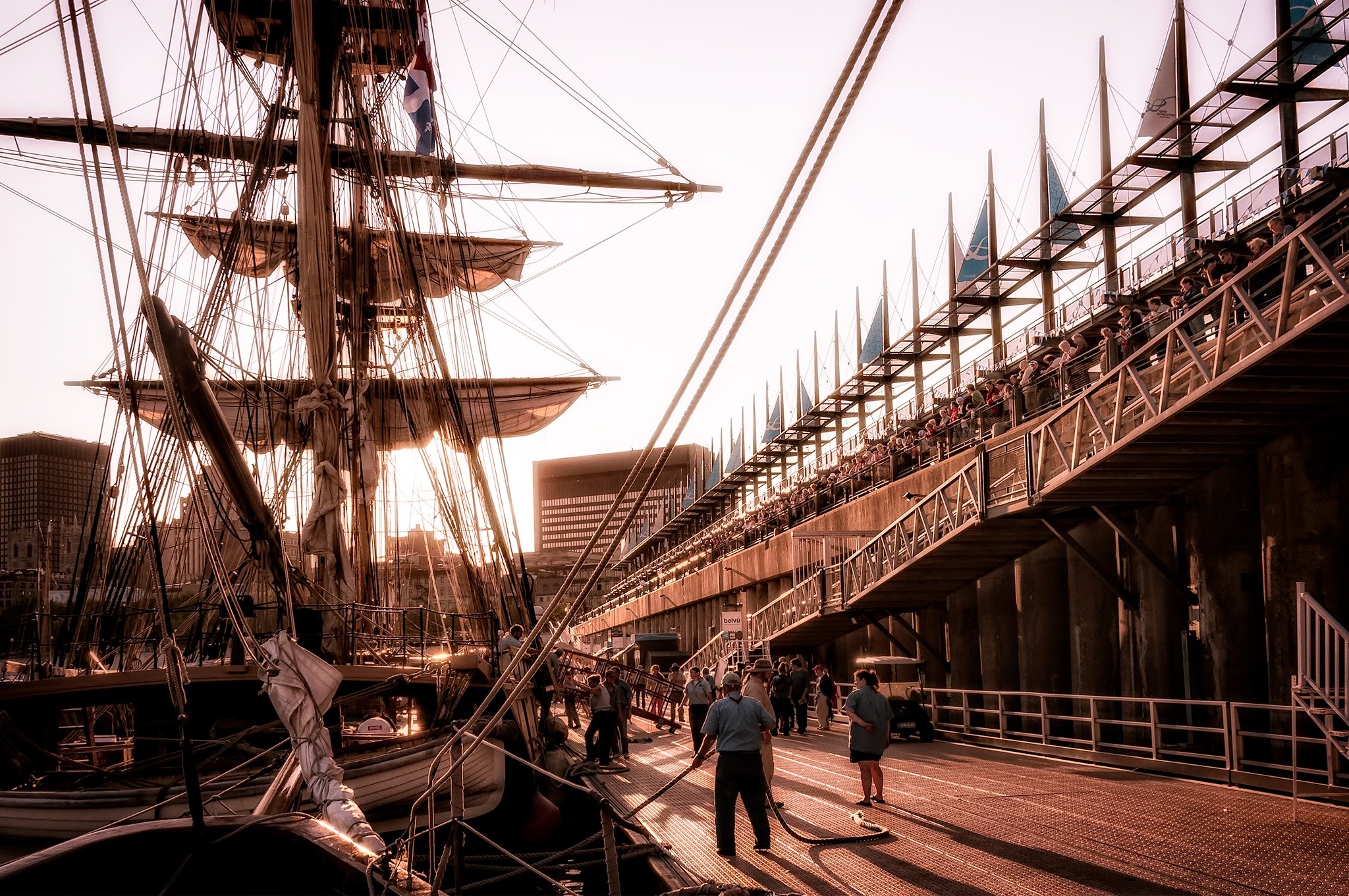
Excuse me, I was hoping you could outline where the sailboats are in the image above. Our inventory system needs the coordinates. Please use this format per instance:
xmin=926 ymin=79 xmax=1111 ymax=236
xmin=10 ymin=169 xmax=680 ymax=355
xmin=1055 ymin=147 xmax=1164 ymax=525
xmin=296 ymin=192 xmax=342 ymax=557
xmin=0 ymin=0 xmax=723 ymax=896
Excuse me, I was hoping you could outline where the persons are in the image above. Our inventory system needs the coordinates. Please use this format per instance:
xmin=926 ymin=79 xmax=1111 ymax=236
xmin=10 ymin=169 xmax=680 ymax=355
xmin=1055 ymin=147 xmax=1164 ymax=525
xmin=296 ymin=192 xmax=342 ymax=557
xmin=692 ymin=672 xmax=777 ymax=855
xmin=844 ymin=669 xmax=895 ymax=806
xmin=495 ymin=624 xmax=837 ymax=769
xmin=741 ymin=658 xmax=783 ymax=809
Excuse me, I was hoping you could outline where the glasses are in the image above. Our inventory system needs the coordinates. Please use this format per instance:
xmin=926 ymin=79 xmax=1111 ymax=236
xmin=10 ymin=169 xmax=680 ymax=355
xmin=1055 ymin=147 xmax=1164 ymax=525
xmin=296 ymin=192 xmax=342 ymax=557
xmin=1251 ymin=247 xmax=1255 ymax=250
xmin=589 ymin=682 xmax=594 ymax=685
xmin=1202 ymin=265 xmax=1208 ymax=270
xmin=1219 ymin=256 xmax=1225 ymax=262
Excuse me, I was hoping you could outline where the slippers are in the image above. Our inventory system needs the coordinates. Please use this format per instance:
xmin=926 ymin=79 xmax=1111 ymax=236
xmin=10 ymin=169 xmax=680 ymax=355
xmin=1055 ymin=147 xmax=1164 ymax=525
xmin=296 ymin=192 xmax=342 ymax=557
xmin=870 ymin=796 xmax=885 ymax=803
xmin=856 ymin=800 xmax=873 ymax=806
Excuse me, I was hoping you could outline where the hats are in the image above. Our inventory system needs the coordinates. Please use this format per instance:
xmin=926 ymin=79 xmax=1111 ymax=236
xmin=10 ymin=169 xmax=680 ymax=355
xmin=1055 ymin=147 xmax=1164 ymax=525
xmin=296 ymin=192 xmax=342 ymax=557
xmin=556 ymin=648 xmax=564 ymax=654
xmin=1118 ymin=317 xmax=1125 ymax=324
xmin=606 ymin=671 xmax=616 ymax=677
xmin=721 ymin=671 xmax=741 ymax=685
xmin=1197 ymin=258 xmax=1215 ymax=274
xmin=984 ymin=381 xmax=995 ymax=387
xmin=956 ymin=397 xmax=963 ymax=403
xmin=670 ymin=663 xmax=680 ymax=670
xmin=745 ymin=658 xmax=778 ymax=672
xmin=1291 ymin=204 xmax=1309 ymax=216
xmin=1028 ymin=360 xmax=1038 ymax=365
xmin=812 ymin=665 xmax=823 ymax=670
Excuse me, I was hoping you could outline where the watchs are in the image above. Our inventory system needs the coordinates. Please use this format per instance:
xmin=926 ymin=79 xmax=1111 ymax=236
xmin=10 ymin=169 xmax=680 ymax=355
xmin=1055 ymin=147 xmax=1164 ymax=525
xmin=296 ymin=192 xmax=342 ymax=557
xmin=697 ymin=753 xmax=705 ymax=760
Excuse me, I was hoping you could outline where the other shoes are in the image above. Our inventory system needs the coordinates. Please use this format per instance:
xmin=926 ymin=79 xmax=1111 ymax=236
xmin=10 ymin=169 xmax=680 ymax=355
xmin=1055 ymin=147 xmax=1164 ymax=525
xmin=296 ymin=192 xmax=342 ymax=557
xmin=572 ymin=724 xmax=581 ymax=729
xmin=753 ymin=841 xmax=770 ymax=849
xmin=690 ymin=754 xmax=696 ymax=758
xmin=582 ymin=757 xmax=596 ymax=763
xmin=623 ymin=753 xmax=630 ymax=758
xmin=610 ymin=754 xmax=620 ymax=759
xmin=817 ymin=726 xmax=831 ymax=731
xmin=717 ymin=848 xmax=736 ymax=856
xmin=765 ymin=802 xmax=784 ymax=808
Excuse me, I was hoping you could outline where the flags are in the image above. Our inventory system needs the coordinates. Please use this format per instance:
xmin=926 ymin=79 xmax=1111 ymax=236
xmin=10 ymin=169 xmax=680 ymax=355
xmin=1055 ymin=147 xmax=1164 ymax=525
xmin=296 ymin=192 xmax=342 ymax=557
xmin=402 ymin=1 xmax=437 ymax=157
